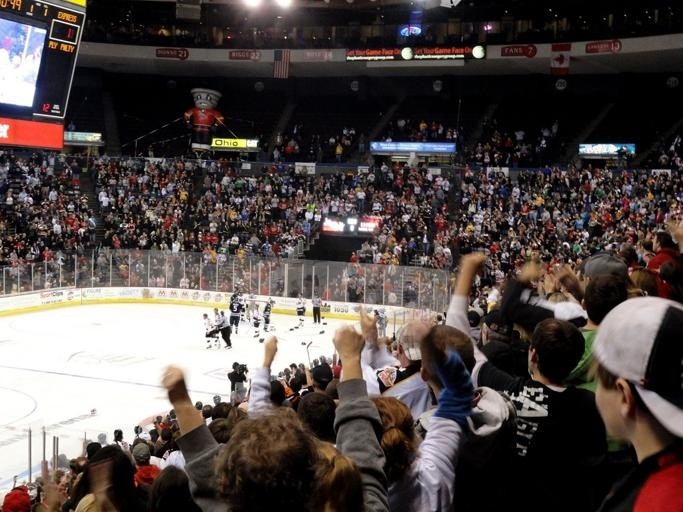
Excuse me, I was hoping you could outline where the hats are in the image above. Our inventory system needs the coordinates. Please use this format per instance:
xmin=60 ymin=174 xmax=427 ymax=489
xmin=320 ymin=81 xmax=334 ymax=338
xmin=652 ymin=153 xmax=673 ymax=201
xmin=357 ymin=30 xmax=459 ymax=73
xmin=585 ymin=252 xmax=643 ymax=295
xmin=309 ymin=365 xmax=331 ymax=382
xmin=591 ymin=296 xmax=683 ymax=429
xmin=133 ymin=443 xmax=150 ymax=462
xmin=3 ymin=485 xmax=38 ymax=512
xmin=480 ymin=309 xmax=513 ymax=346
xmin=395 ymin=322 xmax=429 ymax=361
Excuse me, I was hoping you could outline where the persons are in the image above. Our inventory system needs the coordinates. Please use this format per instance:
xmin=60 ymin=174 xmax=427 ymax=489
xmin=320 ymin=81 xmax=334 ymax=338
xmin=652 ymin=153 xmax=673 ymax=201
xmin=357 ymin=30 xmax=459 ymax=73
xmin=1 ymin=17 xmax=682 ymax=511
